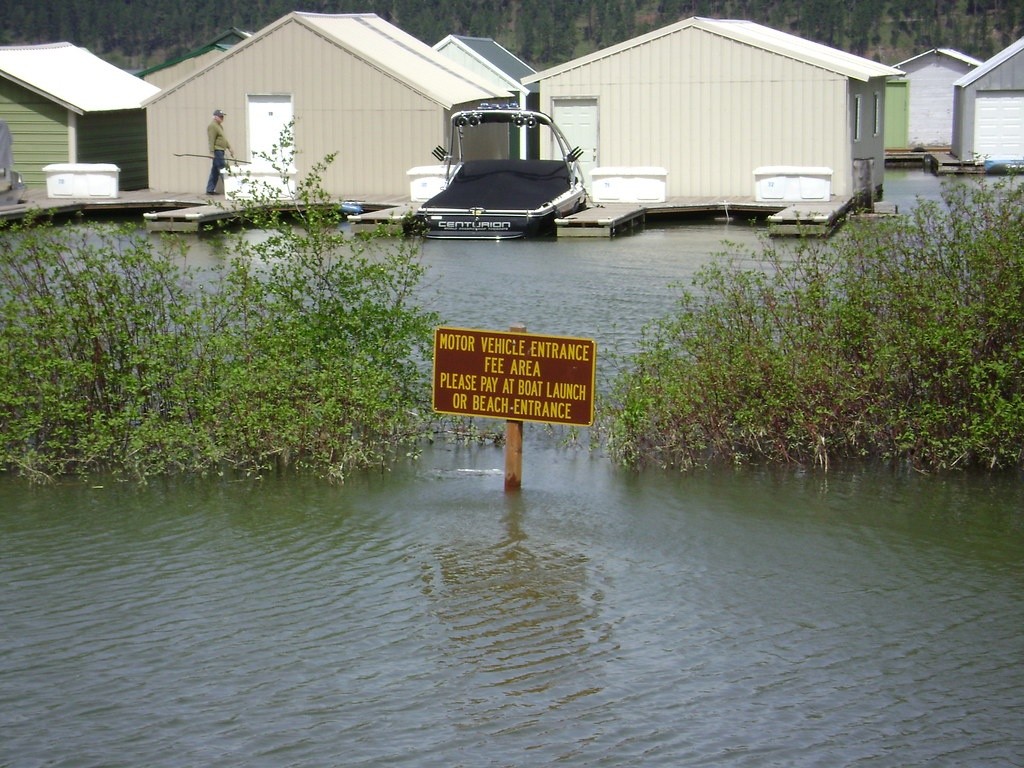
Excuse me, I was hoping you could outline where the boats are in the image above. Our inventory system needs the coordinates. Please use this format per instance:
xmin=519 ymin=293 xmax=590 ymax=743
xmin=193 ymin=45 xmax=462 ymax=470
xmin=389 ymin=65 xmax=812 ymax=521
xmin=402 ymin=101 xmax=589 ymax=242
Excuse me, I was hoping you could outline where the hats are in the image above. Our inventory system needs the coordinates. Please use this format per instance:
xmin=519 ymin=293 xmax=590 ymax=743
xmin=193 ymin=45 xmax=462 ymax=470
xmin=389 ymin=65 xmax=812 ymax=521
xmin=213 ymin=110 xmax=225 ymax=117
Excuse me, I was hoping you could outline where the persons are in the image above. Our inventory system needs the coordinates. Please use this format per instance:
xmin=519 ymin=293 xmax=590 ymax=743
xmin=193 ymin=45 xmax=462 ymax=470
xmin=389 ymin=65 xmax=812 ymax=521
xmin=206 ymin=110 xmax=234 ymax=194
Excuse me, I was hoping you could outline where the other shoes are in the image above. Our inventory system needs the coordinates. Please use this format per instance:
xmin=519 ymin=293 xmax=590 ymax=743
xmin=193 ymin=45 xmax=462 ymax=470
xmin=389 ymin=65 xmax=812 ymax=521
xmin=206 ymin=192 xmax=219 ymax=195
